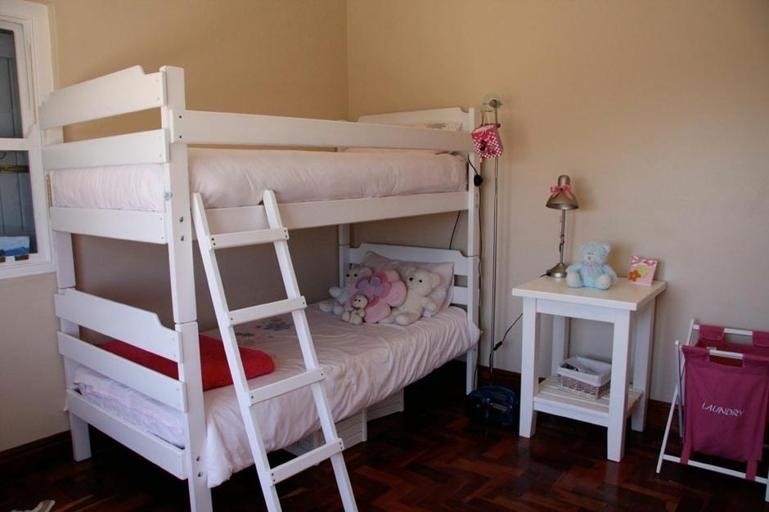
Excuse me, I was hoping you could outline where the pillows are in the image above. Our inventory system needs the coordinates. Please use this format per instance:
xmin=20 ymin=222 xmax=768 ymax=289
xmin=342 ymin=120 xmax=462 ymax=153
xmin=363 ymin=251 xmax=454 ymax=318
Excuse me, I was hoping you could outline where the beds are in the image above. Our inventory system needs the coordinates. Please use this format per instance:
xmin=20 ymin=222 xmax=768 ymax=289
xmin=37 ymin=65 xmax=483 ymax=512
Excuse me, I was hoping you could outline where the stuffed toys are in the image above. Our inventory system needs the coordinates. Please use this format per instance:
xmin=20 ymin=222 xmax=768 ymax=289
xmin=564 ymin=239 xmax=617 ymax=291
xmin=340 ymin=294 xmax=367 ymax=325
xmin=314 ymin=262 xmax=372 ymax=315
xmin=377 ymin=269 xmax=439 ymax=326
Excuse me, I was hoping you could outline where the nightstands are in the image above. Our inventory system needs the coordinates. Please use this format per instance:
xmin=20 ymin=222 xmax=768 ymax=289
xmin=512 ymin=275 xmax=667 ymax=463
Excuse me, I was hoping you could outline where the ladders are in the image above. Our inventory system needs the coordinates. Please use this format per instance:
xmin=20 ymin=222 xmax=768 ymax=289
xmin=192 ymin=190 xmax=358 ymax=511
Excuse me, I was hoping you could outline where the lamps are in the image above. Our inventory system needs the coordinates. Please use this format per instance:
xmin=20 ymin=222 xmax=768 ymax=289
xmin=546 ymin=175 xmax=580 ymax=278
